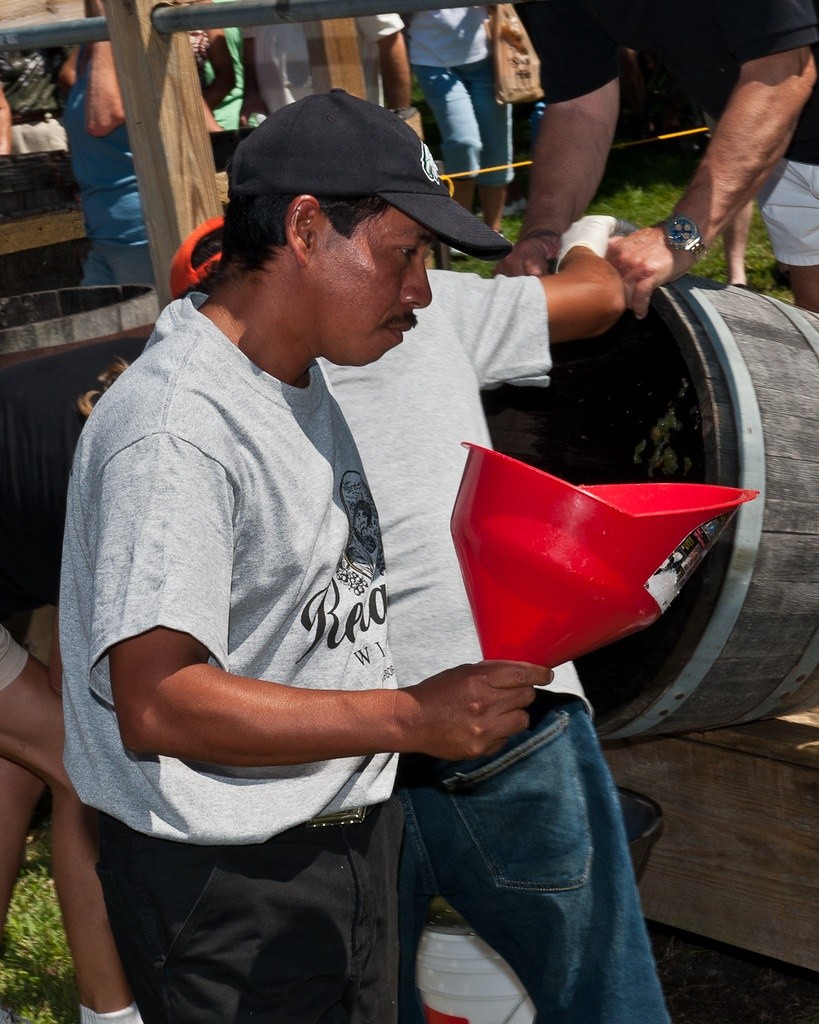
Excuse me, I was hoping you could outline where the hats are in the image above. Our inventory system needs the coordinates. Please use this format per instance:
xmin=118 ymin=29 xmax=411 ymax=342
xmin=169 ymin=215 xmax=224 ymax=300
xmin=226 ymin=88 xmax=514 ymax=262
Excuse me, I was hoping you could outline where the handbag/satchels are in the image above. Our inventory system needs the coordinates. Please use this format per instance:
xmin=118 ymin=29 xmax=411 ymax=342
xmin=492 ymin=2 xmax=544 ymax=102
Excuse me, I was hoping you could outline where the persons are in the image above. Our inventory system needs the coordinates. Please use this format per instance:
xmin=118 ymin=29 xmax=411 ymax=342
xmin=169 ymin=215 xmax=673 ymax=1024
xmin=407 ymin=6 xmax=515 ymax=231
xmin=59 ymin=89 xmax=555 ymax=1024
xmin=240 ymin=13 xmax=412 ymax=128
xmin=0 ymin=84 xmax=11 ymax=154
xmin=190 ymin=0 xmax=258 ymax=130
xmin=0 ymin=620 xmax=145 ymax=1024
xmin=723 ymin=200 xmax=791 ymax=289
xmin=59 ymin=0 xmax=225 ymax=286
xmin=507 ymin=0 xmax=819 ymax=320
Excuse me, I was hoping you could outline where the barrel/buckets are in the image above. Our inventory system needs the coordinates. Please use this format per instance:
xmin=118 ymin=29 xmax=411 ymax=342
xmin=0 ymin=285 xmax=160 ymax=623
xmin=482 ymin=273 xmax=819 ymax=751
xmin=411 ymin=899 xmax=538 ymax=1024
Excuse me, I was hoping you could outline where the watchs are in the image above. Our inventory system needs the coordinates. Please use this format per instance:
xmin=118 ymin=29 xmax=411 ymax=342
xmin=663 ymin=213 xmax=709 ymax=262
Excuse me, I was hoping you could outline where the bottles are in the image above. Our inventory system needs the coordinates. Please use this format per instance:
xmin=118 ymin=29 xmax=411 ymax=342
xmin=248 ymin=113 xmax=267 ymax=127
